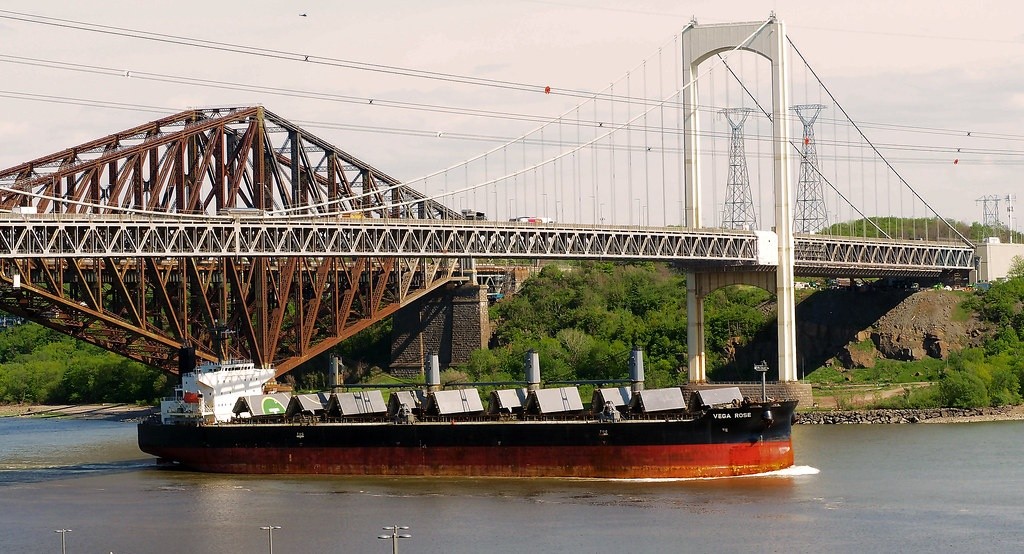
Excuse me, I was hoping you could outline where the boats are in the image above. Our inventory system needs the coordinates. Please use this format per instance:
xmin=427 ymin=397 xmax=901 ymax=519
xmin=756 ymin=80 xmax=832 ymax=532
xmin=136 ymin=321 xmax=800 ymax=475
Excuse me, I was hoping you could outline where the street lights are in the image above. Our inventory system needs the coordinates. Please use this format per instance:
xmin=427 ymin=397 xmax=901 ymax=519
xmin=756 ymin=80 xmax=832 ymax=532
xmin=259 ymin=524 xmax=282 ymax=554
xmin=377 ymin=524 xmax=412 ymax=554
xmin=54 ymin=528 xmax=72 ymax=554
